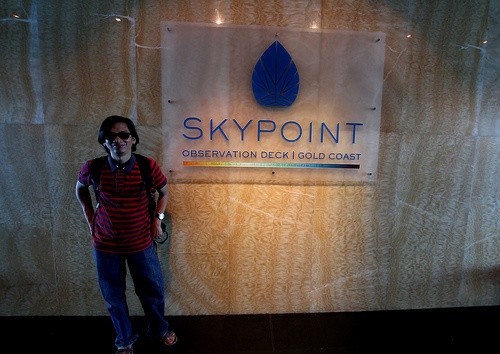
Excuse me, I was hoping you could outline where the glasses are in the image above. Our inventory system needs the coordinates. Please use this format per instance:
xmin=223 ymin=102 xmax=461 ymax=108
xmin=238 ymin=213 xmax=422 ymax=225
xmin=105 ymin=130 xmax=131 ymax=140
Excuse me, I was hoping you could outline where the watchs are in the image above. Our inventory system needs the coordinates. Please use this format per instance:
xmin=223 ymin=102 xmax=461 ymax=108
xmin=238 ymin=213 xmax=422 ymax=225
xmin=155 ymin=212 xmax=165 ymax=221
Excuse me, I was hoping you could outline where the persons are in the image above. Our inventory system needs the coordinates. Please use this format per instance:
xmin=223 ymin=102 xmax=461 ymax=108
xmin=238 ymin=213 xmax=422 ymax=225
xmin=75 ymin=116 xmax=177 ymax=351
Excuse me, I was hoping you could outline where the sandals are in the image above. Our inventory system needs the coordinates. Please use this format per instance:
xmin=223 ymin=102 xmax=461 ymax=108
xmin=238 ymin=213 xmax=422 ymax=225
xmin=116 ymin=343 xmax=133 ymax=354
xmin=160 ymin=330 xmax=178 ymax=346
